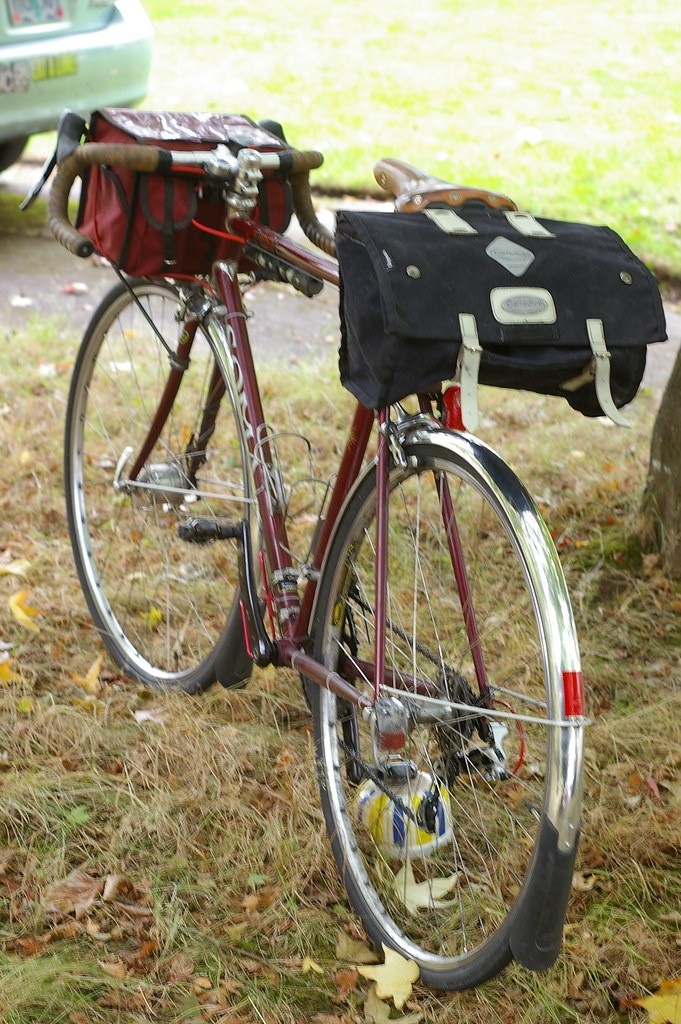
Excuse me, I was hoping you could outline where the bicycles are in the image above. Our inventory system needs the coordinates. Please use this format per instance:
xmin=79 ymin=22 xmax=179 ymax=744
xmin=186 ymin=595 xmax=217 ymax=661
xmin=19 ymin=111 xmax=592 ymax=990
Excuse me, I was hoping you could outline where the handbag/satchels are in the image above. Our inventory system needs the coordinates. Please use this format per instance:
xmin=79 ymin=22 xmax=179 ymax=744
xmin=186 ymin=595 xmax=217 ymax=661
xmin=74 ymin=107 xmax=296 ymax=278
xmin=339 ymin=209 xmax=667 ymax=420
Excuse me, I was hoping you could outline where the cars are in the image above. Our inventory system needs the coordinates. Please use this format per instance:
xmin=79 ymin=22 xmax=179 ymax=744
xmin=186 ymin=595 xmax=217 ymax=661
xmin=2 ymin=1 xmax=151 ymax=169
